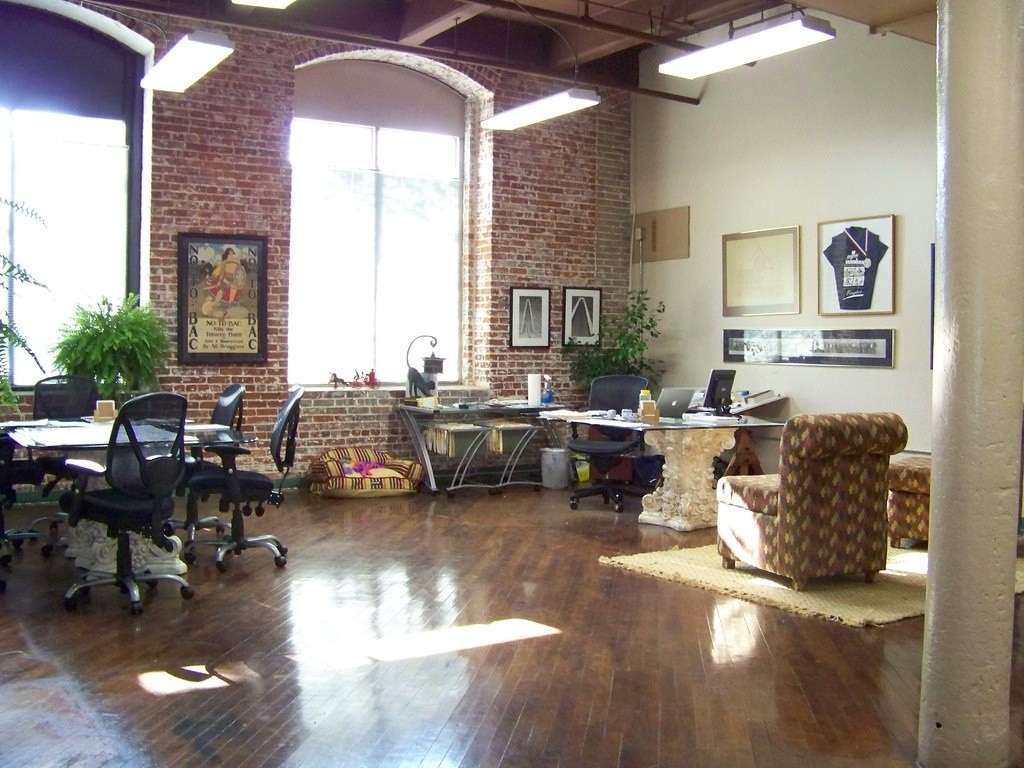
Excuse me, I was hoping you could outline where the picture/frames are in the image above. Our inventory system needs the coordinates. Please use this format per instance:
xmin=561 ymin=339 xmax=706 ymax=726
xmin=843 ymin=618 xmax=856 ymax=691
xmin=817 ymin=214 xmax=896 ymax=316
xmin=509 ymin=286 xmax=551 ymax=349
xmin=722 ymin=226 xmax=800 ymax=317
xmin=176 ymin=231 xmax=267 ymax=364
xmin=562 ymin=286 xmax=603 ymax=348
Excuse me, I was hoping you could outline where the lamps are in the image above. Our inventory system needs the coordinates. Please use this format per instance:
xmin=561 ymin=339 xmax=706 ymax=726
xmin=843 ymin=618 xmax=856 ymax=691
xmin=140 ymin=0 xmax=235 ymax=93
xmin=659 ymin=0 xmax=836 ymax=80
xmin=479 ymin=1 xmax=601 ymax=131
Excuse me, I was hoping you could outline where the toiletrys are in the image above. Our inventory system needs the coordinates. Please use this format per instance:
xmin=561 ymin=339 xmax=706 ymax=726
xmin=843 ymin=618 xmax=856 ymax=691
xmin=541 ymin=374 xmax=554 ymax=404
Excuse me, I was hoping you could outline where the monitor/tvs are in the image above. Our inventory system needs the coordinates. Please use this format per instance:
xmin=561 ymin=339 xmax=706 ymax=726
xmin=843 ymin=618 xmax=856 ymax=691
xmin=702 ymin=369 xmax=736 ymax=416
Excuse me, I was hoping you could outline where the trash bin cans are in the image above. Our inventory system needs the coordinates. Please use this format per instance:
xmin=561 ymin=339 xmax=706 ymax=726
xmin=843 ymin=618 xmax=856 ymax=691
xmin=541 ymin=448 xmax=570 ymax=489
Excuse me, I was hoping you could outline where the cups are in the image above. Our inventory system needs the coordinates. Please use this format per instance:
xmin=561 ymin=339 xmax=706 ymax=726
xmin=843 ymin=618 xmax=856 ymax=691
xmin=622 ymin=409 xmax=632 ymax=418
xmin=608 ymin=409 xmax=616 ymax=420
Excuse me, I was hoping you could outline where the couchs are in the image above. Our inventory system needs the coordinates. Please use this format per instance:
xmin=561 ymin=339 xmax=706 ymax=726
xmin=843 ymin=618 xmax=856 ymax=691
xmin=716 ymin=412 xmax=907 ymax=591
xmin=886 ymin=458 xmax=931 ymax=549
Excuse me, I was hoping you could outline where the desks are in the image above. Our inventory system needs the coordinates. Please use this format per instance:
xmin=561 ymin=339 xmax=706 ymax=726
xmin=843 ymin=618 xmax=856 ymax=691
xmin=394 ymin=402 xmax=579 ymax=497
xmin=0 ymin=417 xmax=259 ymax=577
xmin=535 ymin=412 xmax=785 ymax=532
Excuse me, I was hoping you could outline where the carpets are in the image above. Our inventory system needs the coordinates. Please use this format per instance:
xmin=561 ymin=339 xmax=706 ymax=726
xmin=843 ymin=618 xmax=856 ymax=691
xmin=598 ymin=544 xmax=1024 ymax=628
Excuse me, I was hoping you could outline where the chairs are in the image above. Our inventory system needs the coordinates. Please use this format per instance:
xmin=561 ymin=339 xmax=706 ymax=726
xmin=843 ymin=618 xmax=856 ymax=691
xmin=0 ymin=373 xmax=305 ymax=616
xmin=568 ymin=375 xmax=655 ymax=513
xmin=305 ymin=448 xmax=423 ymax=499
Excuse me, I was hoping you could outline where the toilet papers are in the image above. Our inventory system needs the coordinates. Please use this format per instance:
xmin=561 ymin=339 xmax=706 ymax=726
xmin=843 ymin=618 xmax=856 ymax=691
xmin=528 ymin=374 xmax=541 ymax=408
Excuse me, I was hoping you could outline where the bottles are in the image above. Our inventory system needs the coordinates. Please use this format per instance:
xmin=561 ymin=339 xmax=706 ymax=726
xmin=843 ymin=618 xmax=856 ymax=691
xmin=542 ymin=373 xmax=555 ymax=406
xmin=577 ymin=455 xmax=590 ymax=482
xmin=638 ymin=389 xmax=651 ymax=410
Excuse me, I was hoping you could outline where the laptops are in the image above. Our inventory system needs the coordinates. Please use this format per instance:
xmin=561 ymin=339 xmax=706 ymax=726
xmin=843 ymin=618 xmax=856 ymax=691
xmin=654 ymin=388 xmax=695 ymax=418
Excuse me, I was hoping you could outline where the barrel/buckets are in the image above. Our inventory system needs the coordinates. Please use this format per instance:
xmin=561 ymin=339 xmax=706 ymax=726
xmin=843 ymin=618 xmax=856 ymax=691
xmin=541 ymin=447 xmax=570 ymax=489
